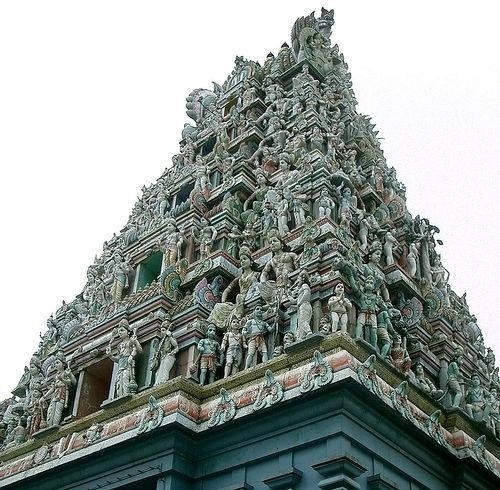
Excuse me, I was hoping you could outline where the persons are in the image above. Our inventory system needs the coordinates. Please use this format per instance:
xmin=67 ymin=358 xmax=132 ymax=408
xmin=0 ymin=6 xmax=500 ymax=448
